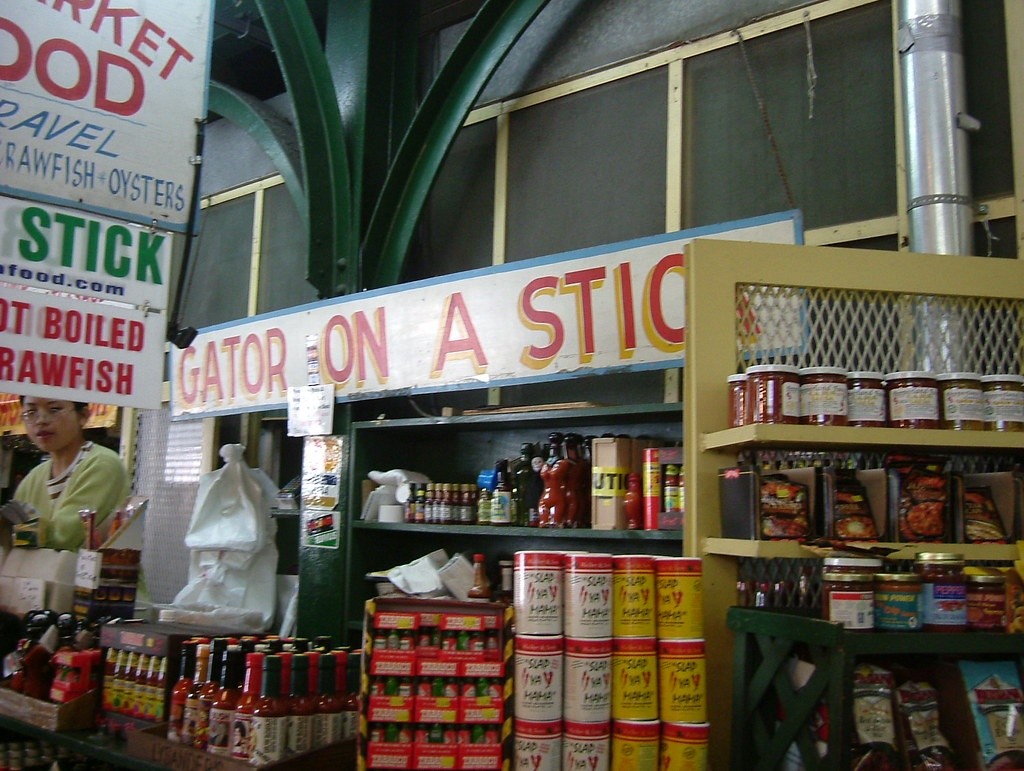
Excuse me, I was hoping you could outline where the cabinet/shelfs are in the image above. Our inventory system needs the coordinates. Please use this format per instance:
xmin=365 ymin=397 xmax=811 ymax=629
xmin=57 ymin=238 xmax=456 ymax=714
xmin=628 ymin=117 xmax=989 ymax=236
xmin=349 ymin=402 xmax=684 ymax=650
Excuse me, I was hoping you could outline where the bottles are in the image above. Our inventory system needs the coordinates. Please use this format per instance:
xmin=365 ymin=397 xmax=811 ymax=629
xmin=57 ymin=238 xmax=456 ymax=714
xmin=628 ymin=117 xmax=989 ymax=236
xmin=369 ymin=630 xmax=501 ymax=744
xmin=99 ymin=623 xmax=362 ymax=769
xmin=404 ymin=433 xmax=650 ymax=529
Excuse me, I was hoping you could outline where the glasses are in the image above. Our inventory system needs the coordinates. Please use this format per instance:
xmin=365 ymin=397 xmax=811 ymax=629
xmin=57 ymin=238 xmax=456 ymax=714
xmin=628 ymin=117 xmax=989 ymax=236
xmin=21 ymin=406 xmax=76 ymax=424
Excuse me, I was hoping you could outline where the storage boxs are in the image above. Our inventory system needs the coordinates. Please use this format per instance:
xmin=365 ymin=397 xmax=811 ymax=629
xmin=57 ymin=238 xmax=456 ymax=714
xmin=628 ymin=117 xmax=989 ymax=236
xmin=1 ymin=550 xmax=358 ymax=771
xmin=719 ymin=466 xmax=1018 ymax=542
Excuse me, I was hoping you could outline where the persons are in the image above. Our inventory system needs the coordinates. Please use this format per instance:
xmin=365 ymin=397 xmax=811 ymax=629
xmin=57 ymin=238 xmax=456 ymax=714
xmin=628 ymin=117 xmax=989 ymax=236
xmin=0 ymin=394 xmax=152 ymax=621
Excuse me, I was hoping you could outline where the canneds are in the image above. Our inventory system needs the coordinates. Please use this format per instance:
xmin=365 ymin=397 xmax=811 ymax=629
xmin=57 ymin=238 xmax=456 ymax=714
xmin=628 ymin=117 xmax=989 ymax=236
xmin=820 ymin=551 xmax=1005 ymax=633
xmin=514 ymin=548 xmax=710 ymax=771
xmin=726 ymin=364 xmax=1024 ymax=432
